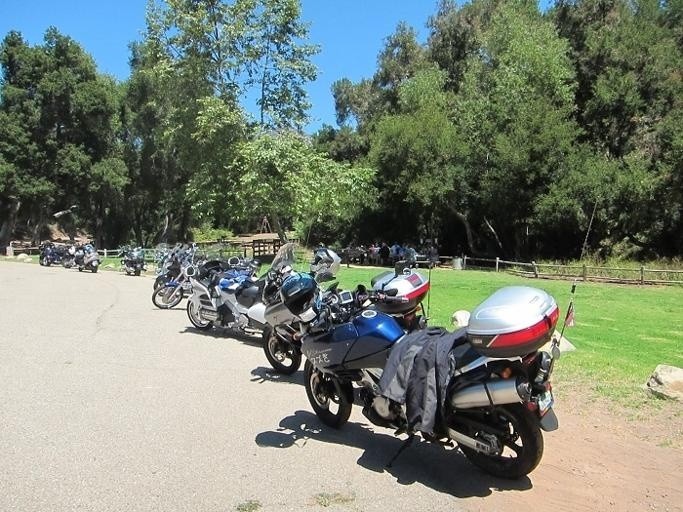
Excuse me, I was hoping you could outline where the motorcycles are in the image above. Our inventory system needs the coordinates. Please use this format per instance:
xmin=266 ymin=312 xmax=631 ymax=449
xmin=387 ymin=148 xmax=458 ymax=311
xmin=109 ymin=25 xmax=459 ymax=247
xmin=151 ymin=242 xmax=431 ymax=374
xmin=117 ymin=239 xmax=147 ymax=276
xmin=38 ymin=238 xmax=103 ymax=274
xmin=287 ymin=259 xmax=573 ymax=480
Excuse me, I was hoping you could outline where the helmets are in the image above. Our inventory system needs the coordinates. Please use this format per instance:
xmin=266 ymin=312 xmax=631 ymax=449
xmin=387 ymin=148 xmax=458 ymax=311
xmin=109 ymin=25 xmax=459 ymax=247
xmin=310 ymin=248 xmax=341 ymax=276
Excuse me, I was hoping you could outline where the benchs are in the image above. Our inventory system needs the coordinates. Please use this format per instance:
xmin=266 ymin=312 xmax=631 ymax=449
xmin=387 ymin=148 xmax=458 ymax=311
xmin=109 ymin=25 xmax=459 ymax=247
xmin=8 ymin=240 xmax=32 ymax=256
xmin=415 ymin=259 xmax=441 ymax=270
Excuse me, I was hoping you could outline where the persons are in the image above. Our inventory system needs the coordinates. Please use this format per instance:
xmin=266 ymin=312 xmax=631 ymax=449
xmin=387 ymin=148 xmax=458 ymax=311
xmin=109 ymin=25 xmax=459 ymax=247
xmin=343 ymin=239 xmax=464 ymax=271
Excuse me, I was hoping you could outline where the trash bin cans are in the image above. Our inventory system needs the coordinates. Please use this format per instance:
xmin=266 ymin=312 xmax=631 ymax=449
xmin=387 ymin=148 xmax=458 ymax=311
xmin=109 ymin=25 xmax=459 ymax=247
xmin=453 ymin=257 xmax=462 ymax=269
